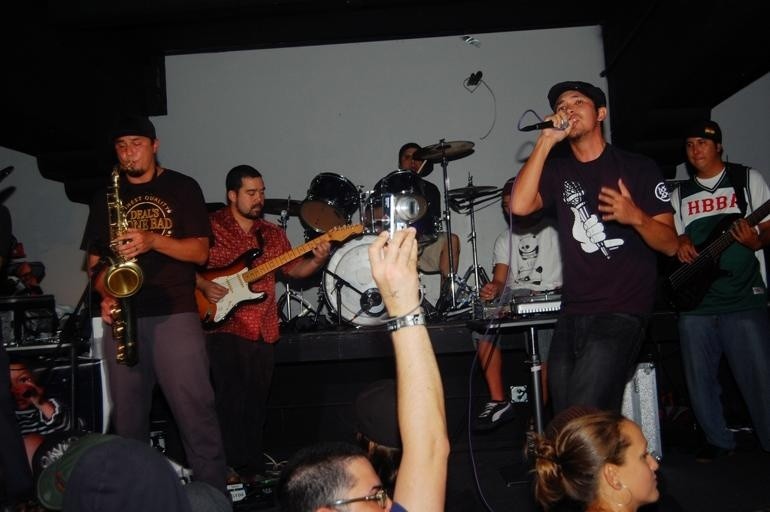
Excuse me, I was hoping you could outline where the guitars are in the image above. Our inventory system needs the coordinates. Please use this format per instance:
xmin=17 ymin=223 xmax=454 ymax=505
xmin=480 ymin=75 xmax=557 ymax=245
xmin=196 ymin=222 xmax=365 ymax=330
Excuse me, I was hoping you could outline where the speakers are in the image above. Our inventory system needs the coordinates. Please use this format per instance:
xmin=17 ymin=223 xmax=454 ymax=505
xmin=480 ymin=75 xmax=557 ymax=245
xmin=27 ymin=356 xmax=103 ymax=433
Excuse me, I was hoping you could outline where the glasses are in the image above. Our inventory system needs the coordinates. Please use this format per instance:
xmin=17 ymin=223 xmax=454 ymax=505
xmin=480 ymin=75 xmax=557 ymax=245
xmin=326 ymin=488 xmax=389 ymax=510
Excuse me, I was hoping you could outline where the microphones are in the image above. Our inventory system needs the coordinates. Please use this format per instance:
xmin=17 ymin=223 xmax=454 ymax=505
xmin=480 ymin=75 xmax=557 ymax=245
xmin=521 ymin=115 xmax=569 ymax=132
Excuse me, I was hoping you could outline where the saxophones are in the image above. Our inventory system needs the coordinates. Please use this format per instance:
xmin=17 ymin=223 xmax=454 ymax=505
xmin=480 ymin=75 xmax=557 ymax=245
xmin=102 ymin=158 xmax=143 ymax=368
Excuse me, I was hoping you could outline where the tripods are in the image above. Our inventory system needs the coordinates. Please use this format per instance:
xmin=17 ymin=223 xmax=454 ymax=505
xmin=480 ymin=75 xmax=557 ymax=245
xmin=456 ymin=200 xmax=491 ymax=309
xmin=435 ymin=151 xmax=474 ymax=313
xmin=274 ymin=209 xmax=331 ymax=327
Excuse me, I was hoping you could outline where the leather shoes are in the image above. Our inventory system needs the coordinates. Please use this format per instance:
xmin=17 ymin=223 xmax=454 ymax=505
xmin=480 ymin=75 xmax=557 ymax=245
xmin=222 ymin=465 xmax=250 ymax=484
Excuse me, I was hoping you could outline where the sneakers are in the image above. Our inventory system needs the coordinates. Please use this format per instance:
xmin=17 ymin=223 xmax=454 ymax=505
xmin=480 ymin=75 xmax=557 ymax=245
xmin=471 ymin=396 xmax=517 ymax=435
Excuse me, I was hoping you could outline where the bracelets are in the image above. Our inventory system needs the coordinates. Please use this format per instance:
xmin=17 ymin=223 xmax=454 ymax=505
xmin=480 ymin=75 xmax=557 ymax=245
xmin=382 ymin=310 xmax=427 ymax=329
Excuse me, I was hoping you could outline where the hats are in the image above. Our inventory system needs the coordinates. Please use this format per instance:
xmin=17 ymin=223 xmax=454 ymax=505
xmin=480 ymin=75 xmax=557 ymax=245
xmin=547 ymin=80 xmax=607 ymax=112
xmin=35 ymin=431 xmax=123 ymax=512
xmin=398 ymin=142 xmax=422 ymax=162
xmin=111 ymin=114 xmax=156 ymax=143
xmin=683 ymin=119 xmax=723 ymax=145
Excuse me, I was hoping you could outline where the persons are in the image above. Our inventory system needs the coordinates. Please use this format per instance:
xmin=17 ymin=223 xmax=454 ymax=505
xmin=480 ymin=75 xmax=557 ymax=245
xmin=277 ymin=227 xmax=450 ymax=511
xmin=363 ymin=144 xmax=460 ymax=310
xmin=508 ymin=78 xmax=680 ymax=417
xmin=674 ymin=119 xmax=768 ymax=486
xmin=10 ymin=361 xmax=71 ymax=468
xmin=523 ymin=407 xmax=663 ymax=510
xmin=473 ymin=176 xmax=562 ymax=434
xmin=197 ymin=165 xmax=331 ymax=487
xmin=30 ymin=427 xmax=233 ymax=511
xmin=82 ymin=113 xmax=230 ymax=495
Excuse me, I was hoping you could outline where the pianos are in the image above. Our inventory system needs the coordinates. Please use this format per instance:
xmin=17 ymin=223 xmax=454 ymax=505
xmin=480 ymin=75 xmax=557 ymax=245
xmin=511 ymin=293 xmax=563 ymax=316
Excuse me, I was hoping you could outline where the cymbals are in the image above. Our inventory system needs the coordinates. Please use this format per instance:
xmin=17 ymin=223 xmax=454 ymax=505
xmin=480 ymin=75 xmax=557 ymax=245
xmin=262 ymin=198 xmax=303 ymax=217
xmin=412 ymin=141 xmax=474 ymax=162
xmin=447 ymin=186 xmax=496 ymax=196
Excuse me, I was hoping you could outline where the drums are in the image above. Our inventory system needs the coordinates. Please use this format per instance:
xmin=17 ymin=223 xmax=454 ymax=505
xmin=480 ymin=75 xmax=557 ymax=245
xmin=301 ymin=171 xmax=361 ymax=232
xmin=374 ymin=167 xmax=436 ymax=244
xmin=321 ymin=226 xmax=426 ymax=327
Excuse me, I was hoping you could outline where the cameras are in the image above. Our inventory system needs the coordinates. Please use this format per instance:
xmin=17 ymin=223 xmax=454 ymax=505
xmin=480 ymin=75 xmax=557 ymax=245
xmin=382 ymin=192 xmax=420 ymax=243
